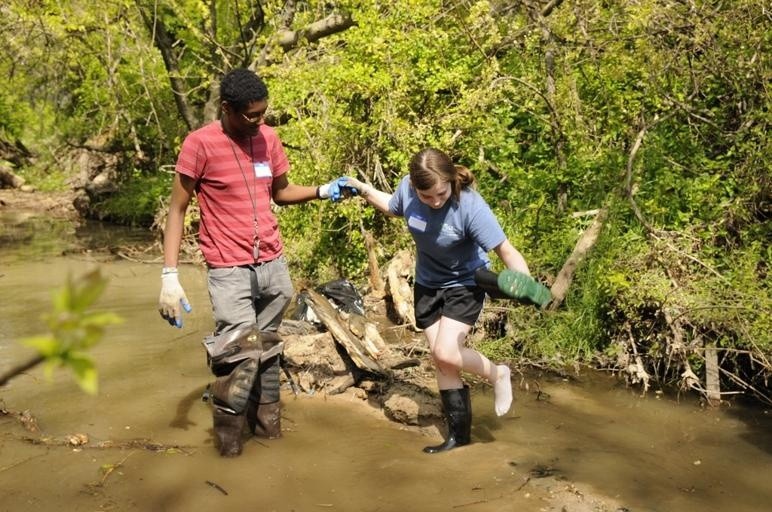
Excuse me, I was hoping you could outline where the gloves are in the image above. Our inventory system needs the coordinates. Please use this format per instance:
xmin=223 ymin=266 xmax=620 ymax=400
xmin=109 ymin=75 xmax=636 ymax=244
xmin=157 ymin=268 xmax=191 ymax=330
xmin=317 ymin=177 xmax=358 ymax=203
xmin=327 ymin=176 xmax=369 ymax=202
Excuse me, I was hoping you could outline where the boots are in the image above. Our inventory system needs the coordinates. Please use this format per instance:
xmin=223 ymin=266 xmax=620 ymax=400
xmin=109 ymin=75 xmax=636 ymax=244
xmin=475 ymin=268 xmax=553 ymax=309
xmin=424 ymin=387 xmax=473 ymax=454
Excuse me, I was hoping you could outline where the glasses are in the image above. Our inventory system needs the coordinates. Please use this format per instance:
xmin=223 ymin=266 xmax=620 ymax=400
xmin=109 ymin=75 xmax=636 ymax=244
xmin=233 ymin=107 xmax=269 ymax=124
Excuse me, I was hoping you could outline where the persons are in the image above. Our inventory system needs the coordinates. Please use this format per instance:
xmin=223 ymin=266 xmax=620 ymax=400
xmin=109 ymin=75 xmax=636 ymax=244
xmin=158 ymin=70 xmax=360 ymax=456
xmin=329 ymin=147 xmax=534 ymax=453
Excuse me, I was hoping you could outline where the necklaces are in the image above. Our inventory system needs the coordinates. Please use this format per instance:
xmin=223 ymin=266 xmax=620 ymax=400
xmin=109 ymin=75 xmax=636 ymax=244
xmin=223 ymin=128 xmax=260 ymax=263
